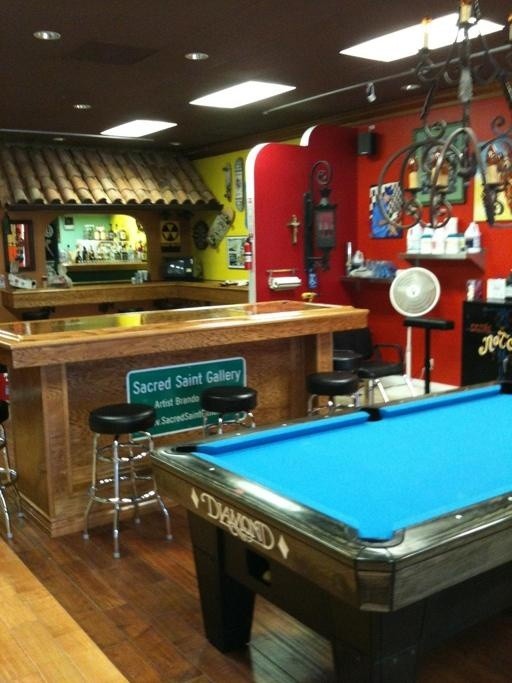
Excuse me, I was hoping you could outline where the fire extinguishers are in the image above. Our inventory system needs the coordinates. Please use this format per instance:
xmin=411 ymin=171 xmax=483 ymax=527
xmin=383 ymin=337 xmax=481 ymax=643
xmin=242 ymin=236 xmax=252 ymax=270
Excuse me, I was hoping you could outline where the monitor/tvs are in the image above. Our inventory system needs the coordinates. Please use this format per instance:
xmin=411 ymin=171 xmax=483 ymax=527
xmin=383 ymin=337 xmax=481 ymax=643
xmin=163 ymin=256 xmax=200 ymax=280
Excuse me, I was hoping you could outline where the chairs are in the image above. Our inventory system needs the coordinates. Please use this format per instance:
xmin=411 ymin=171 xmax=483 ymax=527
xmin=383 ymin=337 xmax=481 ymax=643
xmin=332 ymin=328 xmax=416 ymax=407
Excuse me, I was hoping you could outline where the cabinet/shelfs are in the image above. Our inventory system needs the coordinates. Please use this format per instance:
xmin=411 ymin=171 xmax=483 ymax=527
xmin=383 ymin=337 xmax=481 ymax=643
xmin=399 ymin=249 xmax=486 ymax=275
xmin=462 ymin=299 xmax=512 ymax=386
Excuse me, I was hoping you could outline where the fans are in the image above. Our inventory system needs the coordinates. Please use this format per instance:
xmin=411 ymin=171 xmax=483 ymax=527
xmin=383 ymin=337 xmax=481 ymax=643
xmin=388 ymin=268 xmax=442 ymax=396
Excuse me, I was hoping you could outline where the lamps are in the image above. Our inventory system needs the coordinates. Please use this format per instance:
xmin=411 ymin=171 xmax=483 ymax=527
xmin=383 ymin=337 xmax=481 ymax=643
xmin=304 ymin=160 xmax=339 ymax=291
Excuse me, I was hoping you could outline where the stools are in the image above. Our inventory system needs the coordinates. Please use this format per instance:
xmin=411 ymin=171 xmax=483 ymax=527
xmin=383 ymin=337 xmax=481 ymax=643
xmin=202 ymin=385 xmax=259 ymax=437
xmin=84 ymin=403 xmax=175 ymax=558
xmin=307 ymin=373 xmax=360 ymax=418
xmin=1 ymin=399 xmax=27 ymax=540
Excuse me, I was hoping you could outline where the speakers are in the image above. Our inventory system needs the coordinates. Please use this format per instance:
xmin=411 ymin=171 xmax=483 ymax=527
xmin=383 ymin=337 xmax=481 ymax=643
xmin=355 ymin=129 xmax=377 ymax=159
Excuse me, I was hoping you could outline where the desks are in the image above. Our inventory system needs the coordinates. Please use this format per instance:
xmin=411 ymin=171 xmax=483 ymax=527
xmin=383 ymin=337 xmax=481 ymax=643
xmin=152 ymin=380 xmax=512 ymax=683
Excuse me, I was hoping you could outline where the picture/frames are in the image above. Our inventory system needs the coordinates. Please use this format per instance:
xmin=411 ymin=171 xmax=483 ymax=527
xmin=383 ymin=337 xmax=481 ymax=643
xmin=412 ymin=119 xmax=469 ymax=207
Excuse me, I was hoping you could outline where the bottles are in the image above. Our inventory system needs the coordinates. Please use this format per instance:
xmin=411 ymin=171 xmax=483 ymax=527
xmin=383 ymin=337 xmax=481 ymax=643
xmin=83 ymin=222 xmax=127 ymax=241
xmin=65 ymin=243 xmax=147 ymax=263
